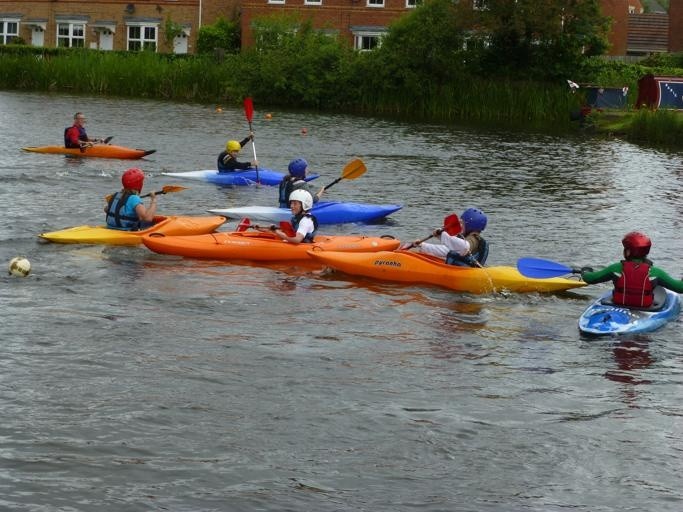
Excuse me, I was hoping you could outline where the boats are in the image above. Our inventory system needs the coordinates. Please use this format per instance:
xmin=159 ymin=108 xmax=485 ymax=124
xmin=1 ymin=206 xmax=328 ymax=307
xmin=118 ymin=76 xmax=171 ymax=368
xmin=20 ymin=145 xmax=155 ymax=159
xmin=38 ymin=216 xmax=226 ymax=245
xmin=304 ymin=246 xmax=589 ymax=295
xmin=205 ymin=201 xmax=404 ymax=226
xmin=577 ymin=287 xmax=679 ymax=339
xmin=161 ymin=169 xmax=320 ymax=186
xmin=140 ymin=230 xmax=399 ymax=260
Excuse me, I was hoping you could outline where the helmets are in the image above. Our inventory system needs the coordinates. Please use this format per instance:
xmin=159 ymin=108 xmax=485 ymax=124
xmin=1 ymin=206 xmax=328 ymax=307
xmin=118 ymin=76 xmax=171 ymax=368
xmin=622 ymin=232 xmax=651 ymax=258
xmin=288 ymin=189 xmax=312 ymax=211
xmin=122 ymin=168 xmax=143 ymax=190
xmin=288 ymin=159 xmax=307 ymax=178
xmin=460 ymin=208 xmax=487 ymax=234
xmin=226 ymin=140 xmax=241 ymax=155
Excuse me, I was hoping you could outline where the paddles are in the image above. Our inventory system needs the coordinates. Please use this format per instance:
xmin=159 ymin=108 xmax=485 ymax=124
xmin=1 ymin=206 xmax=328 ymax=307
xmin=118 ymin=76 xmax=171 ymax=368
xmin=245 ymin=99 xmax=261 ymax=183
xmin=518 ymin=257 xmax=587 ymax=278
xmin=237 ymin=217 xmax=295 ymax=238
xmin=80 ymin=136 xmax=114 ymax=153
xmin=105 ymin=185 xmax=189 ymax=202
xmin=324 ymin=159 xmax=367 ymax=190
xmin=403 ymin=215 xmax=463 ymax=251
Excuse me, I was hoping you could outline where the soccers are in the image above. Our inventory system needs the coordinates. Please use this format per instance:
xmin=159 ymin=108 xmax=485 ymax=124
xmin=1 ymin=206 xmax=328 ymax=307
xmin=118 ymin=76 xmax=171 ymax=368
xmin=9 ymin=256 xmax=31 ymax=277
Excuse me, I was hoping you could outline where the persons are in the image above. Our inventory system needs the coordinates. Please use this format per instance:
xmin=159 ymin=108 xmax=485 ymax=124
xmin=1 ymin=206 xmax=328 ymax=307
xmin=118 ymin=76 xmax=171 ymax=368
xmin=580 ymin=232 xmax=683 ymax=310
xmin=106 ymin=168 xmax=156 ymax=231
xmin=279 ymin=156 xmax=325 ymax=208
xmin=622 ymin=83 xmax=629 ymax=104
xmin=252 ymin=189 xmax=318 ymax=245
xmin=218 ymin=132 xmax=259 ymax=175
xmin=413 ymin=208 xmax=489 ymax=267
xmin=64 ymin=111 xmax=104 ymax=150
